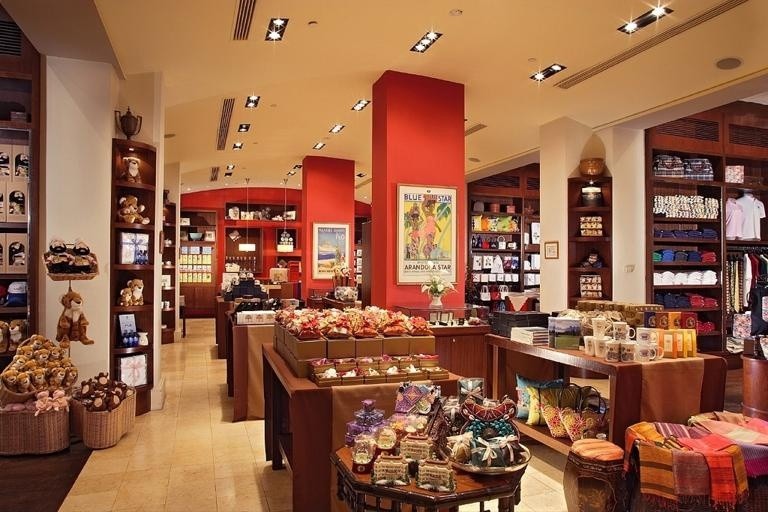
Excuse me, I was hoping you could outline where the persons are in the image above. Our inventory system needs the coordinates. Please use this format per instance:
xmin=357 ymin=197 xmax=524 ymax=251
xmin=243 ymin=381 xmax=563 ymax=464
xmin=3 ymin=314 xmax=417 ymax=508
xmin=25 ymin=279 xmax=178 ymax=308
xmin=725 ymin=197 xmax=746 ymax=240
xmin=420 ymin=198 xmax=443 ymax=260
xmin=408 ymin=201 xmax=423 ymax=260
xmin=736 ymin=194 xmax=766 ymax=241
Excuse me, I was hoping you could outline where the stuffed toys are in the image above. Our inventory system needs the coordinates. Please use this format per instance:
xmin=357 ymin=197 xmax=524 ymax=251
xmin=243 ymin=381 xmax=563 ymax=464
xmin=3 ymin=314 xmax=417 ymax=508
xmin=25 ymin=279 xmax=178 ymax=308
xmin=43 ymin=237 xmax=75 ymax=274
xmin=120 ymin=155 xmax=143 ymax=186
xmin=116 ymin=287 xmax=134 ymax=306
xmin=56 ymin=290 xmax=94 ymax=348
xmin=117 ymin=194 xmax=150 ymax=225
xmin=70 ymin=239 xmax=97 ymax=276
xmin=0 ymin=318 xmax=135 ymax=413
xmin=127 ymin=278 xmax=145 ymax=307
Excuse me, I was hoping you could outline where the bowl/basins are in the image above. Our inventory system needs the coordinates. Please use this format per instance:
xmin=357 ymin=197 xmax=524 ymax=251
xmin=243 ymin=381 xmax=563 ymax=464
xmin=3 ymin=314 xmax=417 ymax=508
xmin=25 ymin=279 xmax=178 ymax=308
xmin=189 ymin=233 xmax=202 ymax=241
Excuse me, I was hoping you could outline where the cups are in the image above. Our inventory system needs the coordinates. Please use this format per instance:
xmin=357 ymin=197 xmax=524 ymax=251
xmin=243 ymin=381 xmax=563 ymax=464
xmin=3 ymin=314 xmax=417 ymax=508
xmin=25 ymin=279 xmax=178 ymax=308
xmin=583 ymin=317 xmax=665 ymax=363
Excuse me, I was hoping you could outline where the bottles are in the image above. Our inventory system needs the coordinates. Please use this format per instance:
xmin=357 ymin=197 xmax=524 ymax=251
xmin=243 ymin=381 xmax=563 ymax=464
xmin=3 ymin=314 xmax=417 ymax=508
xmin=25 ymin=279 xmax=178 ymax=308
xmin=225 ymin=256 xmax=257 ymax=272
xmin=121 ymin=329 xmax=151 ymax=347
xmin=135 ymin=249 xmax=149 ymax=264
xmin=471 ymin=233 xmax=497 ymax=248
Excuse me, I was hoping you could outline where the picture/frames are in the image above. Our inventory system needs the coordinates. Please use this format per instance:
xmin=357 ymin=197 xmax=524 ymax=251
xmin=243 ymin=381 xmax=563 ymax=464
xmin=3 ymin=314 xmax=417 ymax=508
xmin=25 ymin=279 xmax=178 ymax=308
xmin=544 ymin=241 xmax=559 ymax=259
xmin=396 ymin=182 xmax=459 ymax=286
xmin=311 ymin=221 xmax=350 ymax=280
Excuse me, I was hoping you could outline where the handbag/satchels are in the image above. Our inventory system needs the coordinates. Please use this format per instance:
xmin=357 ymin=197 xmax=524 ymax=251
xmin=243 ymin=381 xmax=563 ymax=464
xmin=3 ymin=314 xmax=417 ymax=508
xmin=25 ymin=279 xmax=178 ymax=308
xmin=539 ymin=382 xmax=605 ymax=441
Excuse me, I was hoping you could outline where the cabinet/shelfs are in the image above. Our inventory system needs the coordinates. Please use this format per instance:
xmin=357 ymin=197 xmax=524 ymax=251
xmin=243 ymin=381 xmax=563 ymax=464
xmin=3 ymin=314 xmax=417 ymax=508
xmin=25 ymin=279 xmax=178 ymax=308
xmin=354 ymin=216 xmax=370 ymax=308
xmin=468 ymin=163 xmax=540 ymax=292
xmin=0 ymin=92 xmax=39 ymax=362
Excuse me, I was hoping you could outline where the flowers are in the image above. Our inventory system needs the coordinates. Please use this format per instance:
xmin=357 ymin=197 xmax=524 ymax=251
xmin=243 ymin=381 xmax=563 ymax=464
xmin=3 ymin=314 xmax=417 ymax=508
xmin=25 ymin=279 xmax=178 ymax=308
xmin=420 ymin=272 xmax=458 ymax=296
